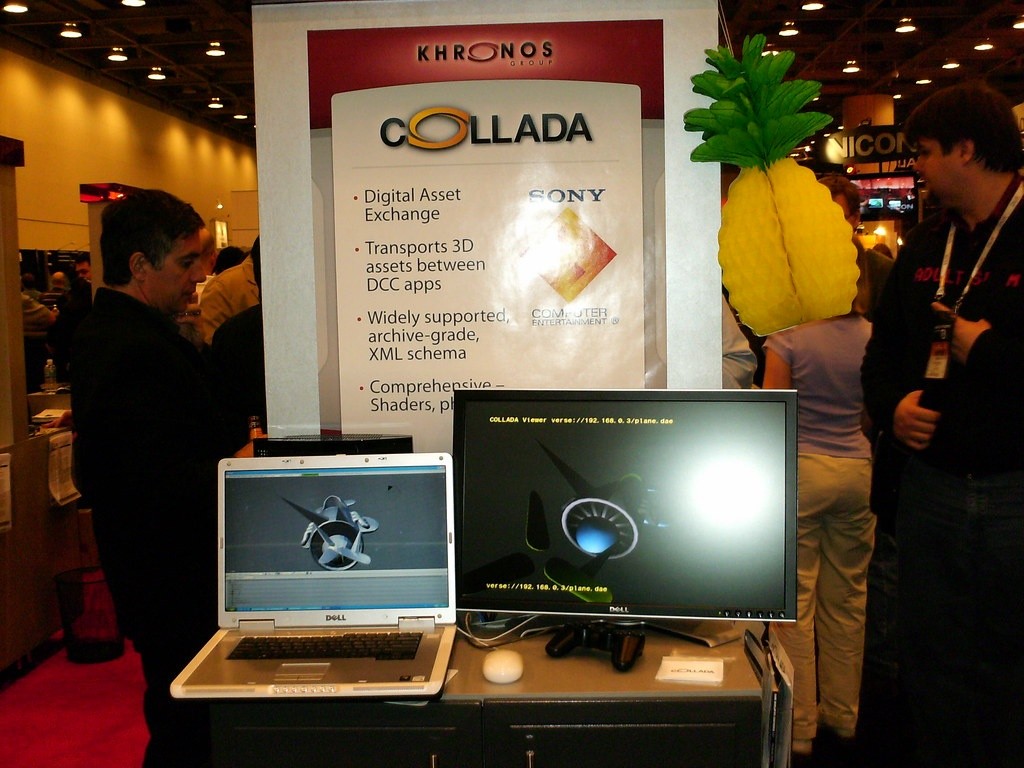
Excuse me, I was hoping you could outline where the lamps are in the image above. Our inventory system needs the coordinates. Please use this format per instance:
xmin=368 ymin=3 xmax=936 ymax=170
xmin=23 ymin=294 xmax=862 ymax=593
xmin=760 ymin=0 xmax=1023 ymax=98
xmin=0 ymin=0 xmax=247 ymax=119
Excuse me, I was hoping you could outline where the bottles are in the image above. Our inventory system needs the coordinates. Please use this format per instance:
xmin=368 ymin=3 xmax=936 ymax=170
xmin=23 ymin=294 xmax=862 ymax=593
xmin=250 ymin=416 xmax=263 ymax=440
xmin=53 ymin=305 xmax=57 ymax=313
xmin=45 ymin=359 xmax=56 ymax=395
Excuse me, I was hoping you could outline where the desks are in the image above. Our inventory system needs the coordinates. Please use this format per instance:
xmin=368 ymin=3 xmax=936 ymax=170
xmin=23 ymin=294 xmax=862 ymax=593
xmin=208 ymin=620 xmax=796 ymax=768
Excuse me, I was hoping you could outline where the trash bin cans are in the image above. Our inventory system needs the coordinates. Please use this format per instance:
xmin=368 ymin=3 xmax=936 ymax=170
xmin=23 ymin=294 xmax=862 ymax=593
xmin=56 ymin=570 xmax=124 ymax=663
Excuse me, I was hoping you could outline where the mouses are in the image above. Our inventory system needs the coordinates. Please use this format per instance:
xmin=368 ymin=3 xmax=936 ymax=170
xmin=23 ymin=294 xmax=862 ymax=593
xmin=483 ymin=648 xmax=524 ymax=683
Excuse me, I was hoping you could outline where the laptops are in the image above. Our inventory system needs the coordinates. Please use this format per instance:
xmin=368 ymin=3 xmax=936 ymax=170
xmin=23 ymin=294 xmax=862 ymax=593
xmin=170 ymin=453 xmax=457 ymax=701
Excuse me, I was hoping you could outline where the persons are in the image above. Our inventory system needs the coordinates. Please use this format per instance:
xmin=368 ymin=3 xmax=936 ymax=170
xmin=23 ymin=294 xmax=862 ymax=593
xmin=857 ymin=83 xmax=1024 ymax=768
xmin=816 ymin=175 xmax=895 ymax=319
xmin=198 ymin=227 xmax=267 ymax=434
xmin=722 ymin=293 xmax=758 ymax=389
xmin=859 ymin=195 xmax=870 ymax=214
xmin=20 ymin=252 xmax=92 ymax=426
xmin=763 ymin=239 xmax=872 ymax=754
xmin=67 ymin=190 xmax=267 ymax=768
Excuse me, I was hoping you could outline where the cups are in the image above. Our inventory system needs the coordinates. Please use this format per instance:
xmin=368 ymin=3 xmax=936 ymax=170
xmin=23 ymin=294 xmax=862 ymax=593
xmin=857 ymin=225 xmax=865 ymax=236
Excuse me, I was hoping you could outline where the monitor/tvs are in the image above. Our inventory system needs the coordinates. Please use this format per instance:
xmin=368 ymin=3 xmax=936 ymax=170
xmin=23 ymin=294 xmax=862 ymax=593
xmin=453 ymin=387 xmax=800 ymax=649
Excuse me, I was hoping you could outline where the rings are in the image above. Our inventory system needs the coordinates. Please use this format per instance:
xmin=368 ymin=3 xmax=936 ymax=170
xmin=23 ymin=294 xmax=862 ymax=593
xmin=185 ymin=313 xmax=190 ymax=315
